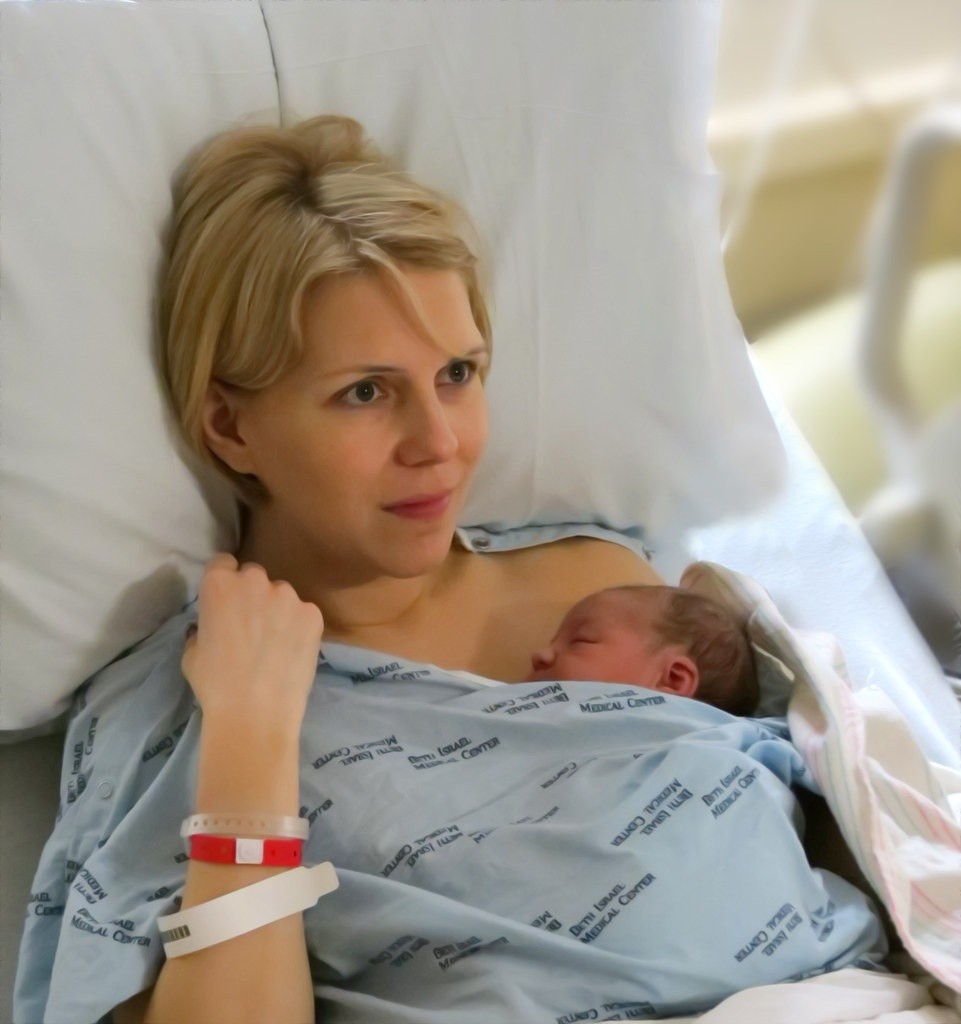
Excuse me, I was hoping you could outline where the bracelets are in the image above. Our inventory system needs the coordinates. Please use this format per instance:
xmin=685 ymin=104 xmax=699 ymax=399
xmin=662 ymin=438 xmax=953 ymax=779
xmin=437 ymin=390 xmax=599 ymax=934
xmin=154 ymin=810 xmax=340 ymax=961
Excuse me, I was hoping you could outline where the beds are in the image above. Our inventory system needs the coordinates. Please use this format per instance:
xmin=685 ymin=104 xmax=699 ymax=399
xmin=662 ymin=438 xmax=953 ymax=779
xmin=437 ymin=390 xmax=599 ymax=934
xmin=0 ymin=0 xmax=961 ymax=1024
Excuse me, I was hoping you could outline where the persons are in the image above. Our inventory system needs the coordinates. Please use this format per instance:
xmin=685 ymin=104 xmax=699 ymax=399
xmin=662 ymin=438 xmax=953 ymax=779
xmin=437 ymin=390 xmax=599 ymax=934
xmin=18 ymin=114 xmax=958 ymax=1024
xmin=521 ymin=579 xmax=764 ymax=732
